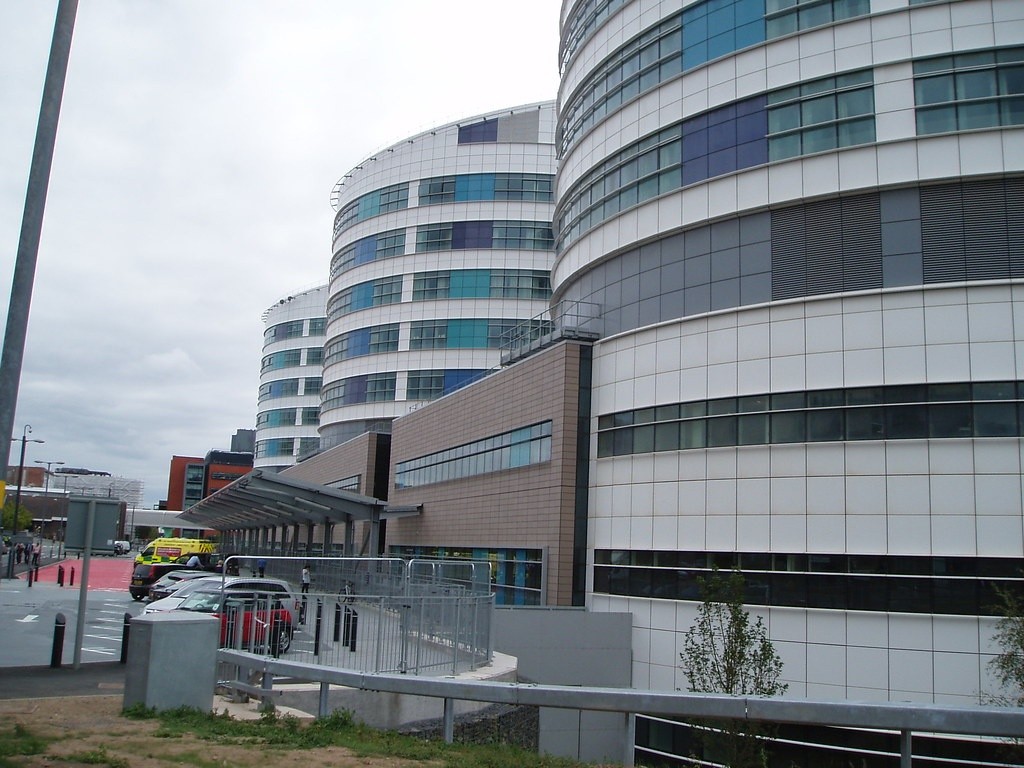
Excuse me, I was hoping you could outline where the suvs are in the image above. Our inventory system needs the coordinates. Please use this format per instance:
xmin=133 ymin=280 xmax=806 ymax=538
xmin=142 ymin=576 xmax=305 ymax=633
xmin=129 ymin=562 xmax=192 ymax=601
xmin=161 ymin=588 xmax=294 ymax=655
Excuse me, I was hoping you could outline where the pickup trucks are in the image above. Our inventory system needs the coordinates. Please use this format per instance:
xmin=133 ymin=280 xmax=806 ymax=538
xmin=147 ymin=552 xmax=239 ymax=580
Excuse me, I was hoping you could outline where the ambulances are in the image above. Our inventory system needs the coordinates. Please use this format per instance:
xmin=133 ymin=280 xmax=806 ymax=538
xmin=134 ymin=536 xmax=218 ymax=572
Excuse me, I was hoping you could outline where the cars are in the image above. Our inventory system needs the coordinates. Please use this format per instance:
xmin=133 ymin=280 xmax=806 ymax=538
xmin=148 ymin=569 xmax=234 ymax=604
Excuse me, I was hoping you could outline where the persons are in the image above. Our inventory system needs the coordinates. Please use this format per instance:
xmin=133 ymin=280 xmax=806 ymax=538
xmin=250 ymin=558 xmax=267 ymax=577
xmin=302 ymin=565 xmax=311 ymax=593
xmin=2 ymin=535 xmax=41 ymax=565
xmin=186 ymin=554 xmax=205 ymax=569
xmin=215 ymin=560 xmax=224 ymax=573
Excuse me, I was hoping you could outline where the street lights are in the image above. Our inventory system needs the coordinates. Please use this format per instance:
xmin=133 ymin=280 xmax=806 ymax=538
xmin=34 ymin=460 xmax=65 ymax=564
xmin=55 ymin=473 xmax=79 ymax=559
xmin=7 ymin=425 xmax=46 ymax=580
